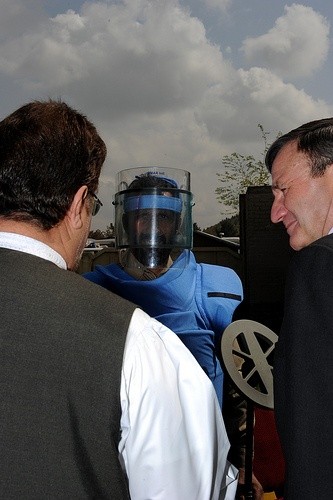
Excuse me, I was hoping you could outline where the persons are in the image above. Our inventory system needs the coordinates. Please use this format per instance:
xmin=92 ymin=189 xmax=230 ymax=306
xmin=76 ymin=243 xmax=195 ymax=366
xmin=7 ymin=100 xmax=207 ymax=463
xmin=80 ymin=165 xmax=257 ymax=443
xmin=238 ymin=116 xmax=333 ymax=500
xmin=0 ymin=101 xmax=241 ymax=500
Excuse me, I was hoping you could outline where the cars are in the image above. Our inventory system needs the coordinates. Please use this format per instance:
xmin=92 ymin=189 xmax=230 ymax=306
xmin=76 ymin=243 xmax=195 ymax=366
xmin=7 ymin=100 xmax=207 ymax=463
xmin=86 ymin=239 xmax=116 ymax=248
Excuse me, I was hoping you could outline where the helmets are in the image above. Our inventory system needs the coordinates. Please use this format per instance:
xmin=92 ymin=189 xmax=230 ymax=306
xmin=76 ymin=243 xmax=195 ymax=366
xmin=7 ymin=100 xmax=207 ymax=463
xmin=112 ymin=167 xmax=193 ymax=270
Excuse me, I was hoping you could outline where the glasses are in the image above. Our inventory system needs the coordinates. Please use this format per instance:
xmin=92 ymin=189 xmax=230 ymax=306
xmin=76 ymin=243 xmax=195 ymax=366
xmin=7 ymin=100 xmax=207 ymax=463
xmin=89 ymin=190 xmax=103 ymax=216
